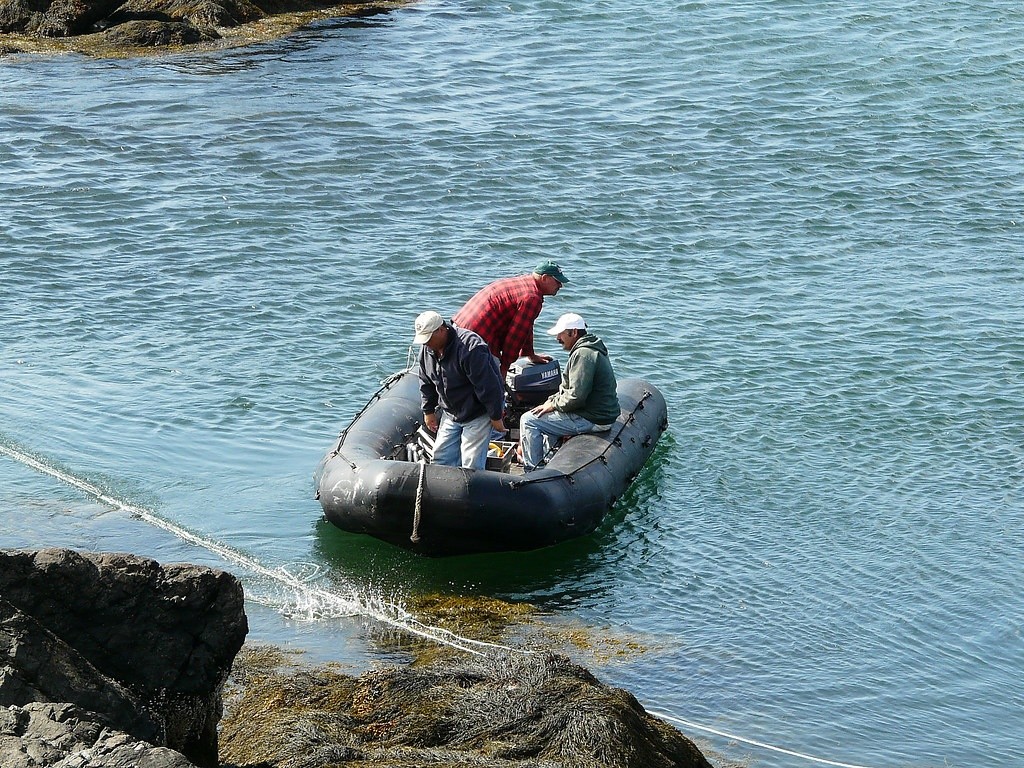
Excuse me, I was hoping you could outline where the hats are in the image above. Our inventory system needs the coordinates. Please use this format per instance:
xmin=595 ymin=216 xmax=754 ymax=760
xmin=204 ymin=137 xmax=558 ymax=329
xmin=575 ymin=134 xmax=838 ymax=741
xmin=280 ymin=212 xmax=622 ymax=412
xmin=547 ymin=313 xmax=588 ymax=335
xmin=413 ymin=311 xmax=443 ymax=344
xmin=535 ymin=260 xmax=571 ymax=283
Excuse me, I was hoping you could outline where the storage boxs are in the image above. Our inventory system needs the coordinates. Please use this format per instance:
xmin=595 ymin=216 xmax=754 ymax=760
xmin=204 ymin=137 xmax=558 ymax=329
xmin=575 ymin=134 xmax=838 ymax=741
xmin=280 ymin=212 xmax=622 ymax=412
xmin=485 ymin=441 xmax=516 ymax=474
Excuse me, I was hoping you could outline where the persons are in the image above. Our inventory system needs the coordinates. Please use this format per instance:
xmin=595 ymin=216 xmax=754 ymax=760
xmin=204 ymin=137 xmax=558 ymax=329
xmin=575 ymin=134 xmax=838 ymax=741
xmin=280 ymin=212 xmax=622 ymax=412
xmin=519 ymin=313 xmax=622 ymax=473
xmin=450 ymin=258 xmax=570 ymax=441
xmin=413 ymin=311 xmax=509 ymax=470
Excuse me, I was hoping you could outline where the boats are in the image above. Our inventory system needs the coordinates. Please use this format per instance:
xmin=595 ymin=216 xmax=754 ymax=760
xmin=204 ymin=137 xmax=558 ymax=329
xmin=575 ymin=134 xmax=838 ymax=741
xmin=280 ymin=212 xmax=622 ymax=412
xmin=314 ymin=357 xmax=673 ymax=557
